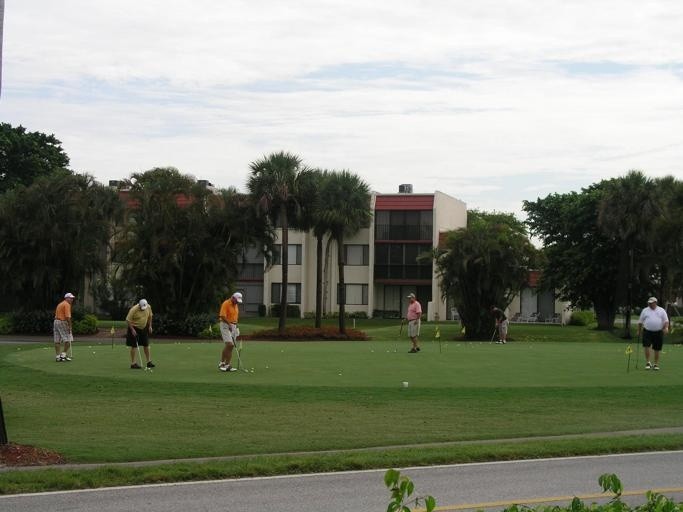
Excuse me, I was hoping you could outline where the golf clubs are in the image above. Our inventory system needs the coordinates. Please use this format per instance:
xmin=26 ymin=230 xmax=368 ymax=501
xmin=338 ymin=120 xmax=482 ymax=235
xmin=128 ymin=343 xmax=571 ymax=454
xmin=135 ymin=335 xmax=148 ymax=369
xmin=230 ymin=331 xmax=248 ymax=372
xmin=489 ymin=325 xmax=497 ymax=345
xmin=69 ymin=330 xmax=76 ymax=360
xmin=391 ymin=320 xmax=405 ymax=353
xmin=635 ymin=336 xmax=640 ymax=371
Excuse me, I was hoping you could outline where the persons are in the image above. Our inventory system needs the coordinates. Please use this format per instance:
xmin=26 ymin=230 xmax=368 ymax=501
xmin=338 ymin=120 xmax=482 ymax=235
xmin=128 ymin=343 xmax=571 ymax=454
xmin=401 ymin=293 xmax=423 ymax=353
xmin=125 ymin=298 xmax=155 ymax=369
xmin=218 ymin=292 xmax=243 ymax=371
xmin=53 ymin=292 xmax=76 ymax=362
xmin=637 ymin=297 xmax=669 ymax=370
xmin=490 ymin=305 xmax=509 ymax=344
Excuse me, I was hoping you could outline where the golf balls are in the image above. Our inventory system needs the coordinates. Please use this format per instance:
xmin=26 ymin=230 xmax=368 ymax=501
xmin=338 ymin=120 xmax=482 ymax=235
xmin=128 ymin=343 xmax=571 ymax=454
xmin=369 ymin=350 xmax=397 ymax=355
xmin=147 ymin=366 xmax=151 ymax=371
xmin=402 ymin=382 xmax=410 ymax=388
xmin=244 ymin=366 xmax=256 ymax=374
xmin=92 ymin=352 xmax=95 ymax=354
xmin=470 ymin=341 xmax=490 ymax=345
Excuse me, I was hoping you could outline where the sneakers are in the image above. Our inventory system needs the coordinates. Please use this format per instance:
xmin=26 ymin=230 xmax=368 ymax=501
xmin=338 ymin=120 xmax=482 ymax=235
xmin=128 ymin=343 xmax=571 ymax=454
xmin=147 ymin=362 xmax=155 ymax=367
xmin=56 ymin=355 xmax=71 ymax=362
xmin=131 ymin=363 xmax=142 ymax=369
xmin=645 ymin=362 xmax=651 ymax=369
xmin=653 ymin=364 xmax=659 ymax=369
xmin=218 ymin=361 xmax=237 ymax=372
xmin=496 ymin=340 xmax=506 ymax=344
xmin=408 ymin=347 xmax=421 ymax=353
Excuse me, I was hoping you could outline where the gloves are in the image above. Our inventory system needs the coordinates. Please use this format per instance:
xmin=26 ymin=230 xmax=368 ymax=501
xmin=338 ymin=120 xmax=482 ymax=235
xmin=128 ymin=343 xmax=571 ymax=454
xmin=236 ymin=327 xmax=239 ymax=336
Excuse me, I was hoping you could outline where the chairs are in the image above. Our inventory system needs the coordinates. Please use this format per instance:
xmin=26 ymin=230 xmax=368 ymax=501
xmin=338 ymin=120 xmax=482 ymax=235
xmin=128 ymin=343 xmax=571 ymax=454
xmin=450 ymin=306 xmax=460 ymax=320
xmin=511 ymin=311 xmax=560 ymax=323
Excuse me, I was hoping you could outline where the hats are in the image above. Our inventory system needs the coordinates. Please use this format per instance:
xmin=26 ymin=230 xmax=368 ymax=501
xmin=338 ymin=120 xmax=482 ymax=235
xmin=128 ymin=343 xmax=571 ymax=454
xmin=405 ymin=293 xmax=415 ymax=299
xmin=64 ymin=293 xmax=75 ymax=299
xmin=648 ymin=296 xmax=658 ymax=303
xmin=139 ymin=299 xmax=148 ymax=310
xmin=233 ymin=292 xmax=243 ymax=302
